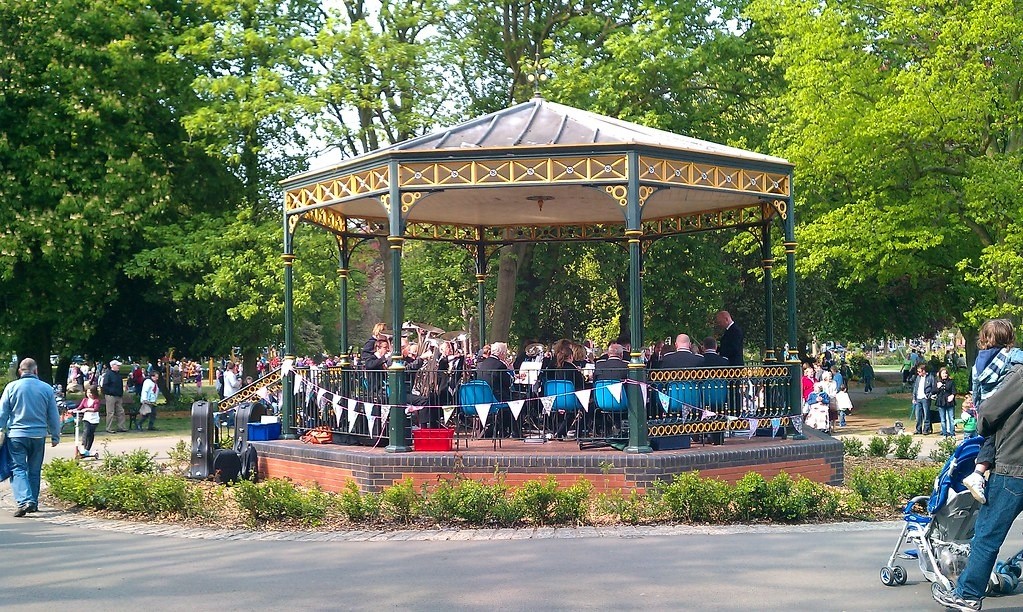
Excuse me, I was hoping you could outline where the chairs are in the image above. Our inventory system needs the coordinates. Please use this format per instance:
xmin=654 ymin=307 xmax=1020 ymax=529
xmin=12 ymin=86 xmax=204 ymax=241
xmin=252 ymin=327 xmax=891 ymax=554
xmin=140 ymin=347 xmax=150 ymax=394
xmin=382 ymin=380 xmax=415 ymax=421
xmin=457 ymin=380 xmax=509 ymax=452
xmin=700 ymin=379 xmax=728 ymax=445
xmin=593 ymin=380 xmax=628 ymax=437
xmin=542 ymin=380 xmax=579 ymax=445
xmin=667 ymin=381 xmax=705 ymax=447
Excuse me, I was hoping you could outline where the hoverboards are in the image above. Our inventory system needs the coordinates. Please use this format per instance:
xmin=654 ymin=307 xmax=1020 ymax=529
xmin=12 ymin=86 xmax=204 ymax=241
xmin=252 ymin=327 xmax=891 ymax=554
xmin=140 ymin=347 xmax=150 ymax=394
xmin=68 ymin=407 xmax=100 ymax=461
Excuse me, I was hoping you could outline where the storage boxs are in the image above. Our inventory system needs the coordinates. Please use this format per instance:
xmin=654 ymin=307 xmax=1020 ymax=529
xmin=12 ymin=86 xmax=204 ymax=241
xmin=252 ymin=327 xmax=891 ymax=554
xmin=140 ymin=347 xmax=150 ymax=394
xmin=412 ymin=428 xmax=454 ymax=451
xmin=756 ymin=427 xmax=784 ymax=437
xmin=649 ymin=435 xmax=690 ymax=451
xmin=246 ymin=422 xmax=282 ymax=441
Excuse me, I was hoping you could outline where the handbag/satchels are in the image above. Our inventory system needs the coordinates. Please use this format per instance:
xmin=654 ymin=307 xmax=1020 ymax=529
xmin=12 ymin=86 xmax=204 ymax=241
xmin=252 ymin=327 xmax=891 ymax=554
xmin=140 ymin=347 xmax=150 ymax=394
xmin=139 ymin=402 xmax=151 ymax=416
xmin=300 ymin=426 xmax=333 ymax=444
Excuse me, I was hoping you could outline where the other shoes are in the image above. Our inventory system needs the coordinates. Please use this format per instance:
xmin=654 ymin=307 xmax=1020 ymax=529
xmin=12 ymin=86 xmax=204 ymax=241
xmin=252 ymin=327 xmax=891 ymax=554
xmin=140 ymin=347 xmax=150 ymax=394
xmin=117 ymin=428 xmax=128 ymax=432
xmin=12 ymin=503 xmax=37 ymax=517
xmin=84 ymin=450 xmax=90 ymax=456
xmin=136 ymin=423 xmax=143 ymax=432
xmin=148 ymin=425 xmax=156 ymax=431
xmin=107 ymin=430 xmax=115 ymax=433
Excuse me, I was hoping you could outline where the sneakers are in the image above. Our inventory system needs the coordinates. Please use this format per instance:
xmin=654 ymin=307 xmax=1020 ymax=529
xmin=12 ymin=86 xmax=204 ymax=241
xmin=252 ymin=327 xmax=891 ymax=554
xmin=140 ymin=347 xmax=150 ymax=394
xmin=930 ymin=580 xmax=983 ymax=612
xmin=963 ymin=473 xmax=986 ymax=505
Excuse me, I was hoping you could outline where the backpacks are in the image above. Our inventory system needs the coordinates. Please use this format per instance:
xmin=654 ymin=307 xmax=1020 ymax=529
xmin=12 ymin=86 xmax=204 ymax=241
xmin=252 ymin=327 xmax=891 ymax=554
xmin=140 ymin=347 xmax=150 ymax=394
xmin=240 ymin=444 xmax=258 ymax=484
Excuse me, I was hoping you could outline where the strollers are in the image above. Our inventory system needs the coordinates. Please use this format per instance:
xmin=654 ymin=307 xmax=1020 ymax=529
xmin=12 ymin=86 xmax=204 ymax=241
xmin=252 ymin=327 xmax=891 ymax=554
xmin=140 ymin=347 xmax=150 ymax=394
xmin=879 ymin=437 xmax=1023 ymax=598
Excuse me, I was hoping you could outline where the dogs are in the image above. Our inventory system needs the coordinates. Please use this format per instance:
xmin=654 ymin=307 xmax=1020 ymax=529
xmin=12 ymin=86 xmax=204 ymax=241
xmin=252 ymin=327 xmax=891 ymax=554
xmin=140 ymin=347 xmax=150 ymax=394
xmin=875 ymin=420 xmax=906 ymax=437
xmin=128 ymin=408 xmax=139 ymax=431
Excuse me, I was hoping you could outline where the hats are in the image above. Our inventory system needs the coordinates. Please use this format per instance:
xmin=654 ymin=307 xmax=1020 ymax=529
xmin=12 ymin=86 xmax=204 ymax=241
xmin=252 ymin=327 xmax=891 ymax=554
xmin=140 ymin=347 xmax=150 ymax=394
xmin=110 ymin=359 xmax=122 ymax=367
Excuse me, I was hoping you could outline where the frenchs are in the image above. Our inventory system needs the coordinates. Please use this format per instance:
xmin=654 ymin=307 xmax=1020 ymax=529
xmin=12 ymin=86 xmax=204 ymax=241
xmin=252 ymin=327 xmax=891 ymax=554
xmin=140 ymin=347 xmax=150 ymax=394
xmin=412 ymin=320 xmax=444 ymax=395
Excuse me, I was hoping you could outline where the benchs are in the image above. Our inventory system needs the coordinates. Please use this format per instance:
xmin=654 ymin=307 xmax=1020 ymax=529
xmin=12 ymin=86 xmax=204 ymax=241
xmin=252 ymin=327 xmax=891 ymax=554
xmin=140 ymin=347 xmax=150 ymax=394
xmin=74 ymin=398 xmax=143 ymax=431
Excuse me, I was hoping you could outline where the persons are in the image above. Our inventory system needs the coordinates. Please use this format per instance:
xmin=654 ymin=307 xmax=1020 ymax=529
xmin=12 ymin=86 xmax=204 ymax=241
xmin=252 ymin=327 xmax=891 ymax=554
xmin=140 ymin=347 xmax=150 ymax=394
xmin=963 ymin=317 xmax=1023 ymax=504
xmin=862 ymin=360 xmax=876 ymax=394
xmin=931 ymin=367 xmax=956 ymax=436
xmin=912 ymin=365 xmax=934 ymax=435
xmin=961 ymin=401 xmax=978 ymax=440
xmin=798 ymin=337 xmax=855 ymax=432
xmin=68 ymin=385 xmax=101 ymax=457
xmin=931 ymin=359 xmax=1023 ymax=612
xmin=900 ymin=347 xmax=967 ymax=387
xmin=102 ymin=360 xmax=130 ymax=432
xmin=135 ymin=371 xmax=162 ymax=431
xmin=67 ymin=322 xmax=764 ymax=444
xmin=0 ymin=358 xmax=61 ymax=517
xmin=717 ymin=311 xmax=743 ymax=430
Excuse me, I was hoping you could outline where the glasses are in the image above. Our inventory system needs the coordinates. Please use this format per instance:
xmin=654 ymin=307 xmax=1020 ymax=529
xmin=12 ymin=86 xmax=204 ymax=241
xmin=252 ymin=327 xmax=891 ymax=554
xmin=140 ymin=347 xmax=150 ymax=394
xmin=917 ymin=370 xmax=923 ymax=373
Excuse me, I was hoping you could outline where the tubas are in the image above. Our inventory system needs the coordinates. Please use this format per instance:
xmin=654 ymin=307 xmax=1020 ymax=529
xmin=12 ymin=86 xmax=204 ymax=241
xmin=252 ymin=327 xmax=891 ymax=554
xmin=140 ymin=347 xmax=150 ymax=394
xmin=441 ymin=330 xmax=472 ymax=383
xmin=379 ymin=330 xmax=411 ymax=362
xmin=525 ymin=342 xmax=545 ymax=357
